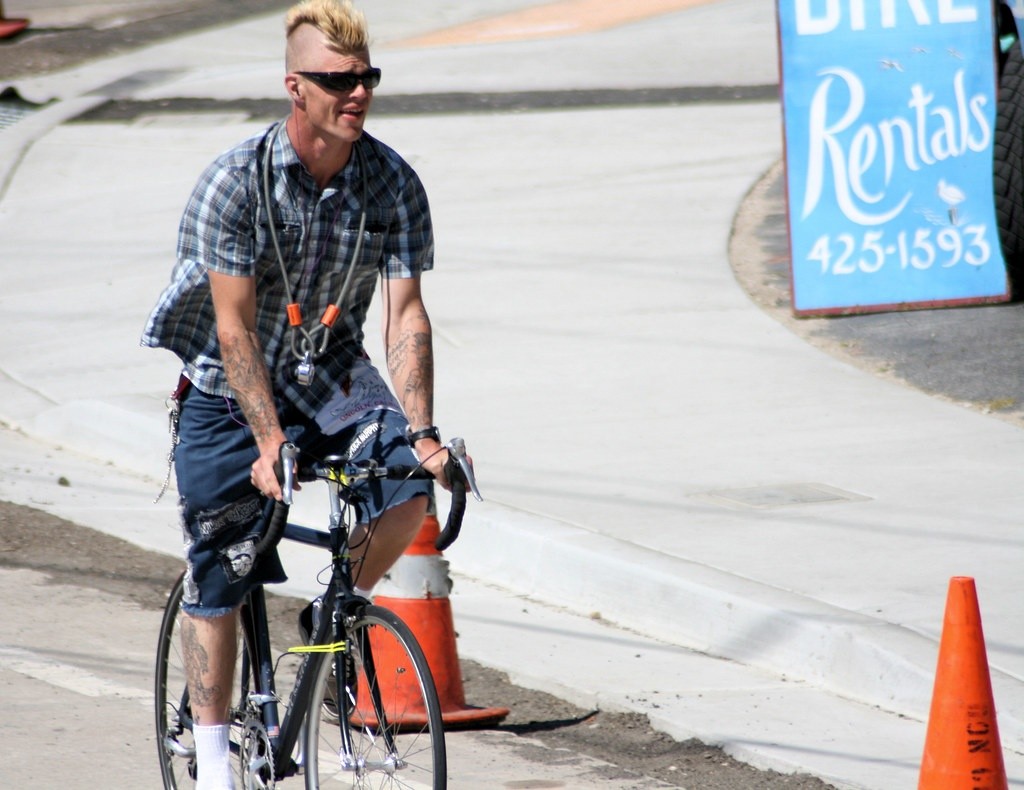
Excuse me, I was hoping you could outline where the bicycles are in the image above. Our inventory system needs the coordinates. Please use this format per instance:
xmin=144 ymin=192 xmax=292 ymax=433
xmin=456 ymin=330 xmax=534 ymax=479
xmin=154 ymin=437 xmax=484 ymax=790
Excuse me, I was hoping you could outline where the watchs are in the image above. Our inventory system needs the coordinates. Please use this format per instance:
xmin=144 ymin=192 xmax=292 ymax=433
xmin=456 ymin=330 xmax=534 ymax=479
xmin=408 ymin=426 xmax=441 ymax=448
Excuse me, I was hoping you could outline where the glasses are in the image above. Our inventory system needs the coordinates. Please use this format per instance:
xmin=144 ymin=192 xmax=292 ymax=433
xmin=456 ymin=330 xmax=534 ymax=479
xmin=295 ymin=67 xmax=382 ymax=91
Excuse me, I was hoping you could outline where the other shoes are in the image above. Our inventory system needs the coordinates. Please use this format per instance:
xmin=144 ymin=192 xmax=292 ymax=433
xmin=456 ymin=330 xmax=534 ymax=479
xmin=297 ymin=599 xmax=358 ymax=721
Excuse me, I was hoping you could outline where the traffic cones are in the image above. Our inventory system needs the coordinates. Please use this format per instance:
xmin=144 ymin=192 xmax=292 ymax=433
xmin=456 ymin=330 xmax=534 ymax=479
xmin=328 ymin=513 xmax=513 ymax=737
xmin=915 ymin=576 xmax=1008 ymax=789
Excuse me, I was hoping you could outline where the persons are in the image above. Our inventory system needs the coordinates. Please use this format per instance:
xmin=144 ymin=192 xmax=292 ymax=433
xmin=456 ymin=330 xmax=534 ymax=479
xmin=139 ymin=0 xmax=473 ymax=790
